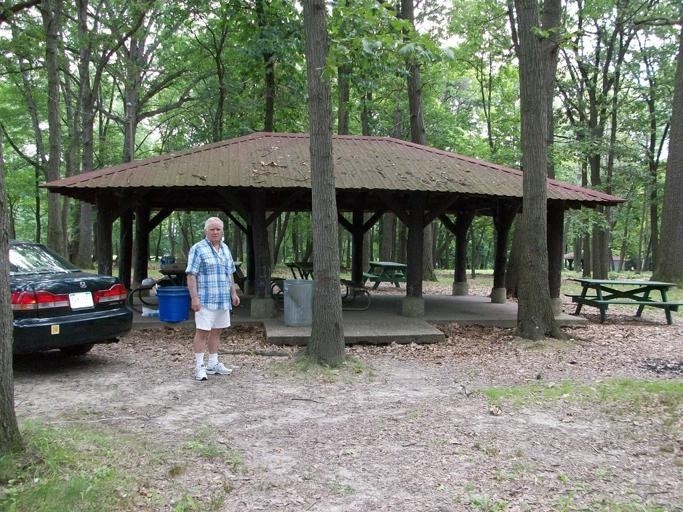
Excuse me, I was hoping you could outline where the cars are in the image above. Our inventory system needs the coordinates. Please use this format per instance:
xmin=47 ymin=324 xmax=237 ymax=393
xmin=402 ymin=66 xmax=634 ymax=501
xmin=9 ymin=240 xmax=133 ymax=359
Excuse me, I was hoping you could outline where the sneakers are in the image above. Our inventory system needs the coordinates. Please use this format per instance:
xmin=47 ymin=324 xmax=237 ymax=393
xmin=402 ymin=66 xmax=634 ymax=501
xmin=206 ymin=362 xmax=233 ymax=375
xmin=191 ymin=363 xmax=208 ymax=381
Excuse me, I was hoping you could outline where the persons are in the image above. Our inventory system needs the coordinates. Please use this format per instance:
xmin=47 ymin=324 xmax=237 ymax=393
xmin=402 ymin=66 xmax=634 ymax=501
xmin=184 ymin=215 xmax=242 ymax=382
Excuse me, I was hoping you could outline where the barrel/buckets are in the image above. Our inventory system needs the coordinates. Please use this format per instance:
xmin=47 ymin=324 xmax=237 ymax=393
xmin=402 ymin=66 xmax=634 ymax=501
xmin=283 ymin=279 xmax=314 ymax=328
xmin=156 ymin=285 xmax=190 ymax=322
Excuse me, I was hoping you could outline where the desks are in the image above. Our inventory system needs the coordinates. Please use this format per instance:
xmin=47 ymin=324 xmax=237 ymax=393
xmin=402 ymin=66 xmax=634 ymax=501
xmin=566 ymin=278 xmax=677 ymax=324
xmin=285 ymin=262 xmax=314 ymax=281
xmin=159 ymin=263 xmax=187 ymax=286
xmin=364 ymin=261 xmax=407 ymax=289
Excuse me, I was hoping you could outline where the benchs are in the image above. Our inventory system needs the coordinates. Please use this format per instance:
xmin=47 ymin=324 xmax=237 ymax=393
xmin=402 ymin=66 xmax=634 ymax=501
xmin=271 ymin=276 xmax=285 ymax=311
xmin=340 ymin=278 xmax=371 ymax=311
xmin=564 ymin=293 xmax=683 ymax=326
xmin=362 ymin=272 xmax=406 ymax=290
xmin=127 ymin=284 xmax=155 ymax=314
